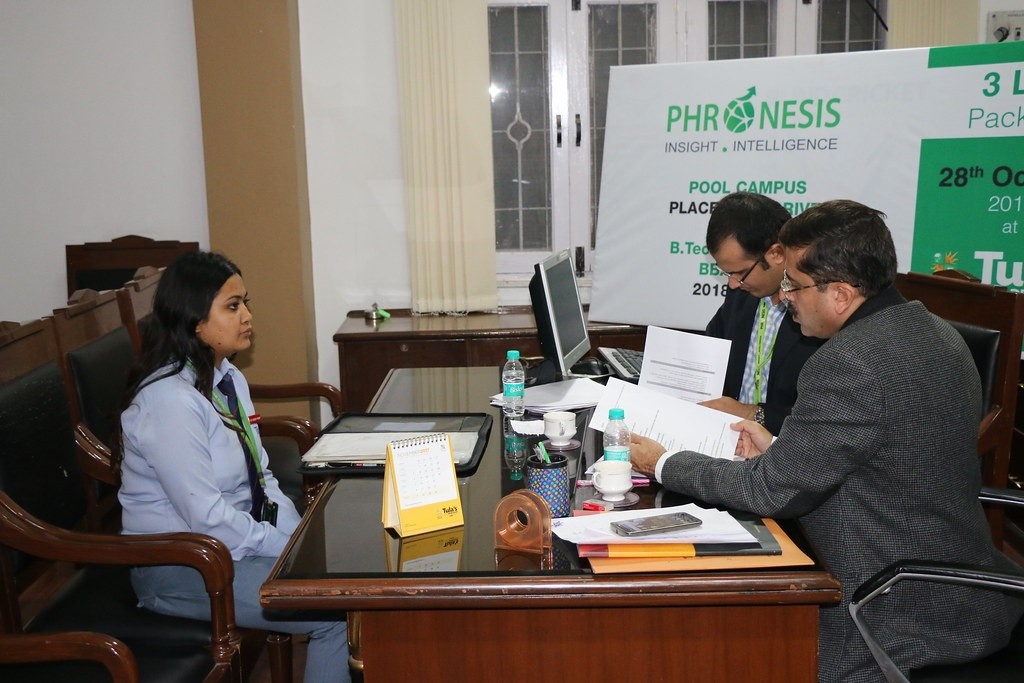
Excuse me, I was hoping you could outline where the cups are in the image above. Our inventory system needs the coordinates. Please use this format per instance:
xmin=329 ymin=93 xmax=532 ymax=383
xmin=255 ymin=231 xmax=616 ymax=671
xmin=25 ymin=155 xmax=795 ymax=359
xmin=590 ymin=460 xmax=633 ymax=501
xmin=543 ymin=411 xmax=577 ymax=447
xmin=525 ymin=453 xmax=570 ymax=518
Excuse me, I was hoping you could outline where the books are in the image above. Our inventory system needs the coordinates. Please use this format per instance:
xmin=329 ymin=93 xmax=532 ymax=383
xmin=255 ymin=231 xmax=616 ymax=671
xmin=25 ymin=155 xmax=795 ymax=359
xmin=574 ymin=509 xmax=815 ymax=576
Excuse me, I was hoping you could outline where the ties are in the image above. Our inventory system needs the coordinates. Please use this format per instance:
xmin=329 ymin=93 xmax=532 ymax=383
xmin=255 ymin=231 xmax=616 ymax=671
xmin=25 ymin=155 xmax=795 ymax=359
xmin=217 ymin=372 xmax=265 ymax=523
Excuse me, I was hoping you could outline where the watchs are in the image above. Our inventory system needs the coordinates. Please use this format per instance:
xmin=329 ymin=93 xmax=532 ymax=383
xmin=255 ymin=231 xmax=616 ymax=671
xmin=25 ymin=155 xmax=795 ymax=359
xmin=754 ymin=404 xmax=765 ymax=426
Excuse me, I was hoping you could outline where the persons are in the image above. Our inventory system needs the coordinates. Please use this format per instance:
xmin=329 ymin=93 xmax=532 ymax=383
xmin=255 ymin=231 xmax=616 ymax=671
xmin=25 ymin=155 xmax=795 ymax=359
xmin=622 ymin=200 xmax=1024 ymax=683
xmin=111 ymin=251 xmax=353 ymax=683
xmin=695 ymin=191 xmax=831 ymax=437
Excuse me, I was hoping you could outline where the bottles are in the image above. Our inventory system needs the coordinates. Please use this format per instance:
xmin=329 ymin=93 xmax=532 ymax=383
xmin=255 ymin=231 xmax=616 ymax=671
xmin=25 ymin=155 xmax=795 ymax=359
xmin=501 ymin=350 xmax=526 ymax=418
xmin=602 ymin=408 xmax=632 ymax=465
xmin=502 ymin=416 xmax=528 ymax=481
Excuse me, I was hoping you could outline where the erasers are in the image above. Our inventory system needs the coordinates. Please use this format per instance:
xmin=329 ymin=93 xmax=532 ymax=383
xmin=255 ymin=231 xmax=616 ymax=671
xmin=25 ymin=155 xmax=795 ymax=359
xmin=583 ymin=498 xmax=614 ymax=511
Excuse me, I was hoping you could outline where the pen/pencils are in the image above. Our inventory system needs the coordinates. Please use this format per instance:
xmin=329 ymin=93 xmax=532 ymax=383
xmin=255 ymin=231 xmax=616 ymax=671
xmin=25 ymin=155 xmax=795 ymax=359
xmin=534 ymin=441 xmax=551 ymax=464
xmin=577 ymin=479 xmax=651 ymax=486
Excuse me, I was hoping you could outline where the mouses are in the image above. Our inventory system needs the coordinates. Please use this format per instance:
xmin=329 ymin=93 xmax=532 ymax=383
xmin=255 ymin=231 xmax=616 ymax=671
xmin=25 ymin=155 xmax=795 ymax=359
xmin=570 ymin=358 xmax=609 ymax=375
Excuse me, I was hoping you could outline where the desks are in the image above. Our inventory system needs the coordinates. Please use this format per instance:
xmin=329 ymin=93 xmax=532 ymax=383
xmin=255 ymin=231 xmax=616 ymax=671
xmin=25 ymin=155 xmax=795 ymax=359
xmin=333 ymin=306 xmax=644 ymax=410
xmin=260 ymin=366 xmax=842 ymax=683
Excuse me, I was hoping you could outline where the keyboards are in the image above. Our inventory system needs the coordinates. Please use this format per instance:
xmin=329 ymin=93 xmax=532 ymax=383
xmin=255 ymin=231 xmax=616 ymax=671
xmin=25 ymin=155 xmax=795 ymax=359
xmin=597 ymin=346 xmax=644 ymax=385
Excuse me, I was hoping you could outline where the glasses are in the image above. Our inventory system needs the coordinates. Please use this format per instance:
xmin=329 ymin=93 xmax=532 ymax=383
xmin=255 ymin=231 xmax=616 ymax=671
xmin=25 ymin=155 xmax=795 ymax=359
xmin=715 ymin=240 xmax=780 ymax=288
xmin=780 ymin=270 xmax=862 ymax=301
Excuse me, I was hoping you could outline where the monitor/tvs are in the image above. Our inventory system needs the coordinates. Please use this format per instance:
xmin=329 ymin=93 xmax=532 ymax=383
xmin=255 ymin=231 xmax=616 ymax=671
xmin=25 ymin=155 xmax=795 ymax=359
xmin=528 ymin=248 xmax=592 ymax=385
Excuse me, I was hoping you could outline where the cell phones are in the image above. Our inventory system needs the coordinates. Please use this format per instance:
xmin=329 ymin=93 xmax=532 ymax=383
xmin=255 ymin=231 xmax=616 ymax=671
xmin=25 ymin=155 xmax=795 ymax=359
xmin=610 ymin=512 xmax=702 ymax=537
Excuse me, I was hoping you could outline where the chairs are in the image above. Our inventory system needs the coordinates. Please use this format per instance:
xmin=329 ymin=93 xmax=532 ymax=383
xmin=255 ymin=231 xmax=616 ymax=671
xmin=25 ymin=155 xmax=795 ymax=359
xmin=905 ymin=270 xmax=1024 ymax=551
xmin=850 ymin=485 xmax=1024 ymax=683
xmin=0 ymin=234 xmax=343 ymax=683
